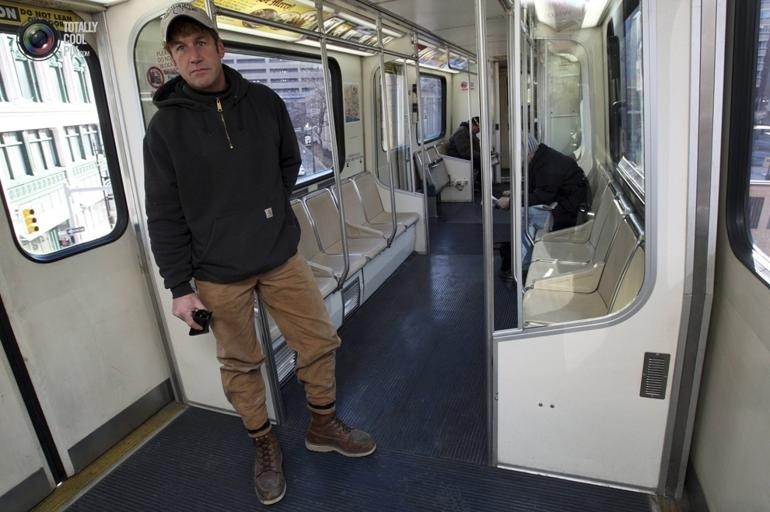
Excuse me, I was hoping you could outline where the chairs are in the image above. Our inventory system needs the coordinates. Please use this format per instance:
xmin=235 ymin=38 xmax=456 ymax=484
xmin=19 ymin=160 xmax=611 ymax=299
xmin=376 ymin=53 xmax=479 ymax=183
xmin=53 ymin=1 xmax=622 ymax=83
xmin=435 ymin=140 xmax=483 ymax=201
xmin=251 ymin=168 xmax=421 ymax=393
xmin=520 ymin=147 xmax=646 ymax=330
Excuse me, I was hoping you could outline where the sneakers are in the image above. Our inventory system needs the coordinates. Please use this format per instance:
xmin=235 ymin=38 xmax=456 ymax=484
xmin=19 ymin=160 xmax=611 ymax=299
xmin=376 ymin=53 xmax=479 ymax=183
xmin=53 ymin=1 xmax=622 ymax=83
xmin=252 ymin=431 xmax=287 ymax=505
xmin=305 ymin=412 xmax=376 ymax=457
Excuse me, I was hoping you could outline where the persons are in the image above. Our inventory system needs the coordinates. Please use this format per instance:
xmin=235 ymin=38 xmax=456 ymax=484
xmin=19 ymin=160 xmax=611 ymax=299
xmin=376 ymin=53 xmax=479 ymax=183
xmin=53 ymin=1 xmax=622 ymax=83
xmin=143 ymin=1 xmax=377 ymax=506
xmin=446 ymin=115 xmax=498 ymax=195
xmin=495 ymin=130 xmax=594 ymax=288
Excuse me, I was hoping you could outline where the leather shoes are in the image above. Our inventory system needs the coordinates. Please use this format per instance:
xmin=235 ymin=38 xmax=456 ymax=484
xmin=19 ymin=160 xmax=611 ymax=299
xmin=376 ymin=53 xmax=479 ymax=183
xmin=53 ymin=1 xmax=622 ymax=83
xmin=498 ymin=271 xmax=513 ymax=280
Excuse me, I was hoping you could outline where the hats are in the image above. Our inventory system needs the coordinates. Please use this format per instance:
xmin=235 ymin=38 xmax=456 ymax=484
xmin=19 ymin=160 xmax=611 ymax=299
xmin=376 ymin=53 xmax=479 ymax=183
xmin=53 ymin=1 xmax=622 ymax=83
xmin=161 ymin=2 xmax=218 ymax=48
xmin=519 ymin=130 xmax=538 ymax=158
xmin=472 ymin=116 xmax=479 ymax=127
